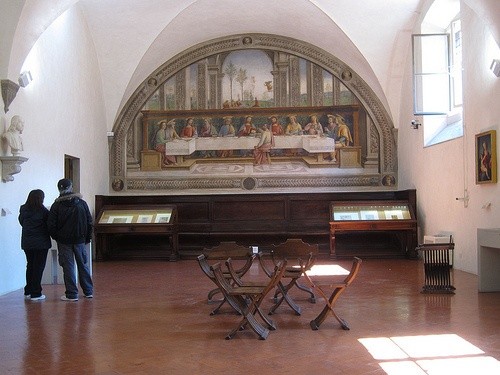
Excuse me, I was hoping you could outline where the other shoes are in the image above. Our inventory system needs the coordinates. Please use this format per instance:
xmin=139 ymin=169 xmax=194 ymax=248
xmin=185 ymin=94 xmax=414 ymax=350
xmin=25 ymin=293 xmax=30 ymax=297
xmin=61 ymin=295 xmax=79 ymax=301
xmin=31 ymin=295 xmax=45 ymax=300
xmin=83 ymin=292 xmax=95 ymax=297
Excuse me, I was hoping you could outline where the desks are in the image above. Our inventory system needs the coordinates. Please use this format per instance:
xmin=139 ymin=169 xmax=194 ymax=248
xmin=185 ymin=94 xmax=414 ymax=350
xmin=476 ymin=228 xmax=500 ymax=293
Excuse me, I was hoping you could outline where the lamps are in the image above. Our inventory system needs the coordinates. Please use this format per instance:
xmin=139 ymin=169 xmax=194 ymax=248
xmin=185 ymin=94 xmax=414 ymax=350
xmin=19 ymin=71 xmax=32 ymax=87
xmin=490 ymin=59 xmax=500 ymax=77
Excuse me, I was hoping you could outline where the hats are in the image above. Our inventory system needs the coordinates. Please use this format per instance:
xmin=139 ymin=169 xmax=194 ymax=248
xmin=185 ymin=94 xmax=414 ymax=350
xmin=57 ymin=178 xmax=73 ymax=191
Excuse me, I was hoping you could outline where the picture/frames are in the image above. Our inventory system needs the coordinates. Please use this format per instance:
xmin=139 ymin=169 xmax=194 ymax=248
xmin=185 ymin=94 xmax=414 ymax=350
xmin=475 ymin=130 xmax=497 ymax=184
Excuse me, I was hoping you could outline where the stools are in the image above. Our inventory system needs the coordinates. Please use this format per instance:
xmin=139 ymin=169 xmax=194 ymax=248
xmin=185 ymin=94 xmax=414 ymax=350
xmin=197 ymin=239 xmax=362 ymax=341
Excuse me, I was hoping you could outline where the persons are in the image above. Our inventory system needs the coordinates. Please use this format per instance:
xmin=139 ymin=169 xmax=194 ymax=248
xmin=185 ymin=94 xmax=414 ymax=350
xmin=49 ymin=179 xmax=94 ymax=301
xmin=19 ymin=189 xmax=51 ymax=300
xmin=5 ymin=115 xmax=25 ymax=155
